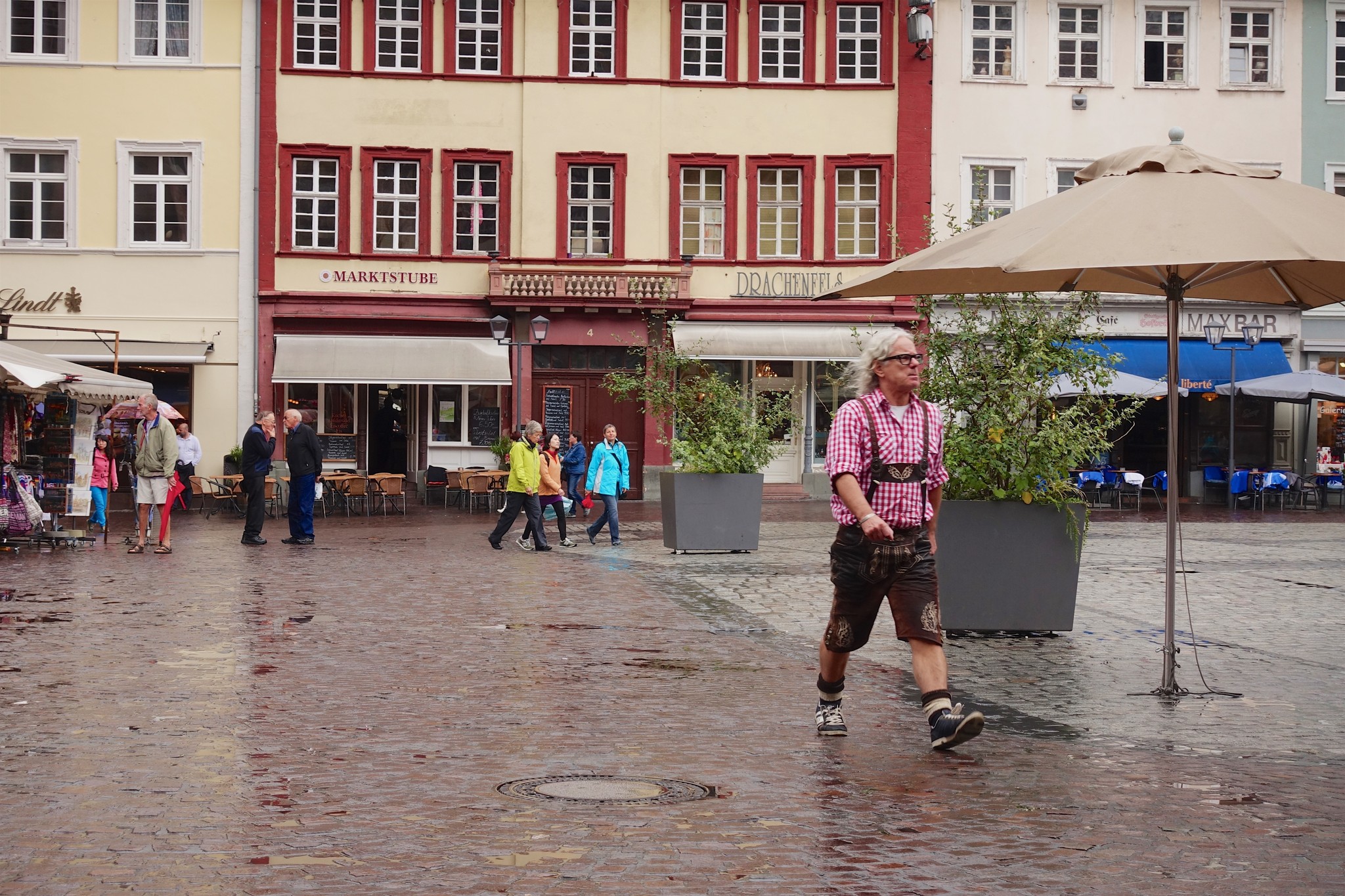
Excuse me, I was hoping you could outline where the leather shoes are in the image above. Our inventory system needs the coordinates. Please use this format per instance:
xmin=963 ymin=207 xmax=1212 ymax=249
xmin=170 ymin=505 xmax=177 ymax=511
xmin=182 ymin=505 xmax=190 ymax=511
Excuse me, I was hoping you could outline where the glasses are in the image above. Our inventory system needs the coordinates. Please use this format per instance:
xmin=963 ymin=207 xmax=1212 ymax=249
xmin=283 ymin=415 xmax=298 ymax=419
xmin=569 ymin=436 xmax=575 ymax=439
xmin=876 ymin=352 xmax=925 ymax=366
xmin=264 ymin=418 xmax=276 ymax=425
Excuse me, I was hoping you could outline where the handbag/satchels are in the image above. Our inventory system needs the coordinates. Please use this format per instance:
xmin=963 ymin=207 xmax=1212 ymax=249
xmin=0 ymin=470 xmax=43 ymax=539
xmin=619 ymin=486 xmax=627 ymax=499
xmin=559 ymin=468 xmax=568 ymax=482
xmin=314 ymin=478 xmax=323 ymax=500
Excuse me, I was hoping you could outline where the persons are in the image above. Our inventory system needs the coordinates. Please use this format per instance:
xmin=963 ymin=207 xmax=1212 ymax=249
xmin=170 ymin=423 xmax=201 ymax=517
xmin=516 ymin=432 xmax=577 ymax=551
xmin=559 ymin=429 xmax=591 ymax=518
xmin=128 ymin=393 xmax=179 ymax=554
xmin=86 ymin=435 xmax=119 ymax=534
xmin=584 ymin=423 xmax=630 ymax=546
xmin=497 ymin=431 xmax=526 ymax=514
xmin=281 ymin=409 xmax=323 ymax=544
xmin=487 ymin=420 xmax=553 ymax=550
xmin=814 ymin=326 xmax=987 ymax=750
xmin=240 ymin=410 xmax=276 ymax=544
xmin=373 ymin=396 xmax=401 ymax=473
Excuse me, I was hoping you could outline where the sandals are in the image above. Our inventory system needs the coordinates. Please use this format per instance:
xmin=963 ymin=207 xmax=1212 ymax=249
xmin=154 ymin=544 xmax=173 ymax=555
xmin=127 ymin=543 xmax=144 ymax=553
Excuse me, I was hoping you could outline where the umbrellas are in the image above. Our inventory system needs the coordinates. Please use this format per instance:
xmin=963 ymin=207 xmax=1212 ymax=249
xmin=1016 ymin=364 xmax=1189 ymax=400
xmin=1214 ymin=369 xmax=1345 ymax=496
xmin=103 ymin=398 xmax=185 ymax=420
xmin=811 ymin=144 xmax=1345 ymax=698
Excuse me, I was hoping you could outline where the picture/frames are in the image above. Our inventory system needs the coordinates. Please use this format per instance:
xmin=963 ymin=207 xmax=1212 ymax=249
xmin=542 ymin=384 xmax=574 ymax=458
xmin=283 ymin=431 xmax=359 ymax=464
xmin=36 ymin=396 xmax=97 ymax=517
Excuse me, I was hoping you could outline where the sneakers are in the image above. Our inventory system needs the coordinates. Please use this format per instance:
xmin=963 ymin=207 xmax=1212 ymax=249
xmin=296 ymin=537 xmax=315 ymax=545
xmin=559 ymin=538 xmax=577 ymax=548
xmin=86 ymin=517 xmax=96 ymax=534
xmin=611 ymin=538 xmax=624 ymax=546
xmin=488 ymin=535 xmax=502 ymax=550
xmin=926 ymin=704 xmax=983 ymax=751
xmin=246 ymin=534 xmax=267 ymax=545
xmin=100 ymin=525 xmax=110 ymax=533
xmin=586 ymin=527 xmax=596 ymax=545
xmin=535 ymin=545 xmax=552 ymax=551
xmin=814 ymin=701 xmax=848 ymax=736
xmin=521 ymin=510 xmax=526 ymax=514
xmin=497 ymin=507 xmax=505 ymax=513
xmin=582 ymin=507 xmax=592 ymax=517
xmin=564 ymin=511 xmax=576 ymax=518
xmin=241 ymin=534 xmax=249 ymax=544
xmin=515 ymin=535 xmax=534 ymax=551
xmin=281 ymin=536 xmax=300 ymax=544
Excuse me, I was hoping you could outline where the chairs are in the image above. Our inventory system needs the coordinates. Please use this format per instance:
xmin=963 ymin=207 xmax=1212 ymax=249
xmin=190 ymin=468 xmax=511 ymax=518
xmin=1029 ymin=458 xmax=1345 ymax=519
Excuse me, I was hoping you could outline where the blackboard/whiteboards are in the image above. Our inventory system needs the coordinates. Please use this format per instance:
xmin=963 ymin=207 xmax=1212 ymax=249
xmin=543 ymin=384 xmax=573 ymax=457
xmin=283 ymin=432 xmax=358 ymax=462
xmin=472 ymin=407 xmax=499 ymax=447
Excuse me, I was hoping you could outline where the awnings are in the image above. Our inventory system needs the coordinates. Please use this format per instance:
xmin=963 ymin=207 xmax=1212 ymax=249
xmin=0 ymin=340 xmax=154 ymax=405
xmin=666 ymin=321 xmax=895 ymax=362
xmin=1040 ymin=338 xmax=1293 ymax=392
xmin=0 ymin=339 xmax=215 ymax=365
xmin=271 ymin=334 xmax=513 ymax=386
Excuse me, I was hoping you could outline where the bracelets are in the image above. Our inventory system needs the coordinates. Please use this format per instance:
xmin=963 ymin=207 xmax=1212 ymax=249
xmin=859 ymin=513 xmax=877 ymax=525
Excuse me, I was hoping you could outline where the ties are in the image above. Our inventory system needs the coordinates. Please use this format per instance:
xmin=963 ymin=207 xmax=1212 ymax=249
xmin=2 ymin=396 xmax=27 ymax=467
xmin=288 ymin=430 xmax=294 ymax=439
xmin=263 ymin=432 xmax=272 ymax=466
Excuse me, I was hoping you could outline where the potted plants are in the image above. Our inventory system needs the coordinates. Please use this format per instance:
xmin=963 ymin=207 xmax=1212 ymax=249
xmin=849 ymin=167 xmax=1141 ymax=639
xmin=598 ymin=311 xmax=801 ymax=558
